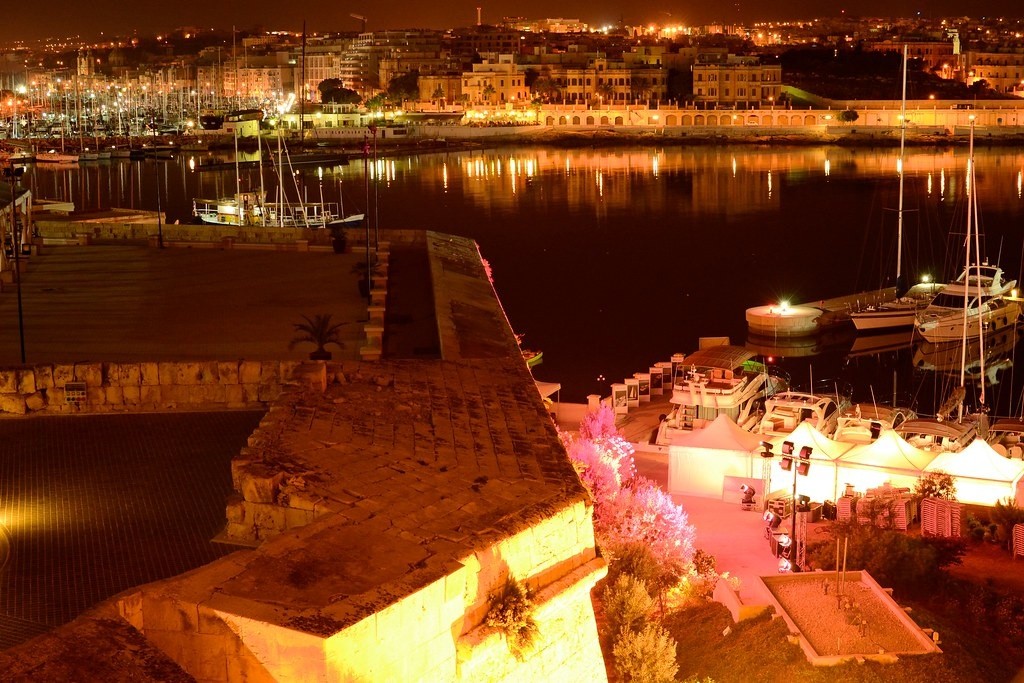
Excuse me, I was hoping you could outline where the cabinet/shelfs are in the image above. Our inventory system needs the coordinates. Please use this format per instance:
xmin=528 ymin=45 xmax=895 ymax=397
xmin=768 ymin=494 xmax=791 ymax=518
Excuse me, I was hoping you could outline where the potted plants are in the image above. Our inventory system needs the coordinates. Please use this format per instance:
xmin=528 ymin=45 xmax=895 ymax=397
xmin=328 ymin=226 xmax=348 ymax=252
xmin=287 ymin=312 xmax=351 ymax=360
xmin=350 ymin=261 xmax=383 ymax=298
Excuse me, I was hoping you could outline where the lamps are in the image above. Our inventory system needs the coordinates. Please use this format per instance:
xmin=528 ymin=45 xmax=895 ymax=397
xmin=795 ymin=492 xmax=811 ymax=513
xmin=740 ymin=483 xmax=756 ymax=509
xmin=759 ymin=441 xmax=774 ymax=458
xmin=763 ymin=509 xmax=782 ymax=531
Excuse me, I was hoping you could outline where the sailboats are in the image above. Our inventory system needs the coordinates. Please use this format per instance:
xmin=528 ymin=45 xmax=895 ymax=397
xmin=1 ymin=25 xmax=299 ymax=139
xmin=910 ymin=325 xmax=1024 ymax=390
xmin=915 ymin=119 xmax=1024 ymax=345
xmin=845 ymin=44 xmax=950 ymax=333
xmin=847 ymin=327 xmax=923 ymax=390
xmin=188 ymin=116 xmax=367 ymax=230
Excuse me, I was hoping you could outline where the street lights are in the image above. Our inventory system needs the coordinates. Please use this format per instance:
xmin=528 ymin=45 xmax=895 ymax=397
xmin=148 ymin=107 xmax=163 ymax=247
xmin=366 ymin=118 xmax=379 ymax=255
xmin=776 ymin=440 xmax=814 ymax=572
xmin=2 ymin=164 xmax=29 ymax=363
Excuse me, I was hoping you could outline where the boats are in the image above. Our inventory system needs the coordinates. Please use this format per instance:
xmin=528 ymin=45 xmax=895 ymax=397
xmin=653 ymin=358 xmax=1024 ymax=464
xmin=744 ymin=333 xmax=850 ymax=365
xmin=745 ymin=281 xmax=898 ymax=340
